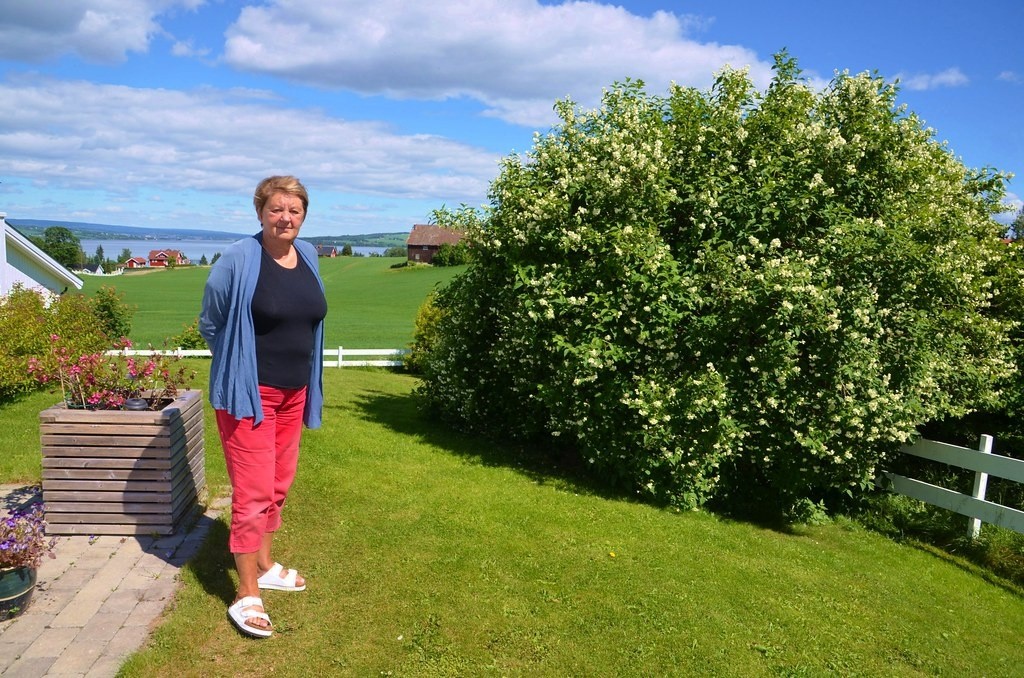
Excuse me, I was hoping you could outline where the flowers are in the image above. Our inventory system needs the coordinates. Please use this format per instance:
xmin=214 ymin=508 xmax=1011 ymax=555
xmin=0 ymin=509 xmax=49 ymax=569
xmin=30 ymin=332 xmax=198 ymax=410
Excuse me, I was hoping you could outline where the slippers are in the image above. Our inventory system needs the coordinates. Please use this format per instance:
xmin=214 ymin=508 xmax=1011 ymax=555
xmin=226 ymin=596 xmax=274 ymax=638
xmin=257 ymin=562 xmax=306 ymax=591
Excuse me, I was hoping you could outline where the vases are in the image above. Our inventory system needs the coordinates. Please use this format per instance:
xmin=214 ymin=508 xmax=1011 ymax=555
xmin=38 ymin=388 xmax=208 ymax=534
xmin=0 ymin=567 xmax=37 ymax=623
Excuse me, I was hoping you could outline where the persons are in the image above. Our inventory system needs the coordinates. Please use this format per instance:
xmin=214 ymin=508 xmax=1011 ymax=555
xmin=199 ymin=176 xmax=328 ymax=639
